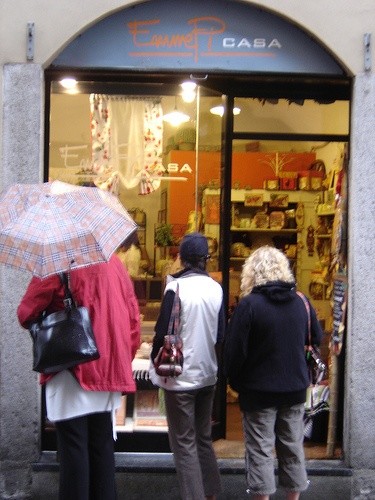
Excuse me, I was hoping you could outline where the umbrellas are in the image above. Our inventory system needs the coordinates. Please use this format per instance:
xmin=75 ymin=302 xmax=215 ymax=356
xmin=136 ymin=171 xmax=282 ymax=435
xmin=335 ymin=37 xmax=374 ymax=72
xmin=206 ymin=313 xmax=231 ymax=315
xmin=0 ymin=181 xmax=138 ymax=279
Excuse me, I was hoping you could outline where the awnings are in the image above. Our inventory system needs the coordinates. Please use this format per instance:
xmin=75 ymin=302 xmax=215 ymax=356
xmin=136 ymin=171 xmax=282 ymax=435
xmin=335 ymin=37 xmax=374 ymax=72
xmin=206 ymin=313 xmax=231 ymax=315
xmin=49 ymin=0 xmax=347 ymax=78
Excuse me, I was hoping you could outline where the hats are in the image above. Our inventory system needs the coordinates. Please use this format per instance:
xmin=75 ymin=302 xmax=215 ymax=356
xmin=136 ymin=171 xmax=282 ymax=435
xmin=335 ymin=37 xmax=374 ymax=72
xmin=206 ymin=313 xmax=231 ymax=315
xmin=179 ymin=233 xmax=209 ymax=256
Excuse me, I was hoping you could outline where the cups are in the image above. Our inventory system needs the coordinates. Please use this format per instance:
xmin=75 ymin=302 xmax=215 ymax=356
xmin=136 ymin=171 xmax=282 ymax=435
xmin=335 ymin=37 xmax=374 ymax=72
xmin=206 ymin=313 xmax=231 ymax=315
xmin=165 ymin=335 xmax=175 ymax=349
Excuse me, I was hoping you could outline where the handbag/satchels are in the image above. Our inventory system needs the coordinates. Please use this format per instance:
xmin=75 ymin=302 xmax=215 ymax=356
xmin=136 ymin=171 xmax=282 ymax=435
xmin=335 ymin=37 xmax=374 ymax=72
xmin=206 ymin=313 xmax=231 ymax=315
xmin=295 ymin=289 xmax=325 ymax=385
xmin=28 ymin=273 xmax=101 ymax=375
xmin=153 ymin=282 xmax=184 ymax=379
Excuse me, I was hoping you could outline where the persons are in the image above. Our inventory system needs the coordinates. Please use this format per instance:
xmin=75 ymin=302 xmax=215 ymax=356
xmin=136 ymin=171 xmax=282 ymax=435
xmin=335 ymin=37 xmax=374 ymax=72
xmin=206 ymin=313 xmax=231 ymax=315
xmin=150 ymin=234 xmax=224 ymax=500
xmin=223 ymin=246 xmax=322 ymax=500
xmin=17 ymin=254 xmax=142 ymax=500
xmin=119 ymin=239 xmax=141 ymax=276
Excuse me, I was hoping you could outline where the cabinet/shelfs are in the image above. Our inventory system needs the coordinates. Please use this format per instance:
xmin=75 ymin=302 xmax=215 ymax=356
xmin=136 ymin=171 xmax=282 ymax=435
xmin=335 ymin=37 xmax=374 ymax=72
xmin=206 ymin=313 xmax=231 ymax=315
xmin=204 ymin=187 xmax=330 ymax=320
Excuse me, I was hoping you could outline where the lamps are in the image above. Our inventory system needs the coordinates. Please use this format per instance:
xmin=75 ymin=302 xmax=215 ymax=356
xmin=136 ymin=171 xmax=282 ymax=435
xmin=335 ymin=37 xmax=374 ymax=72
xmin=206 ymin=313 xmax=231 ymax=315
xmin=163 ymin=96 xmax=189 ymax=126
xmin=210 ymin=95 xmax=241 ymax=117
